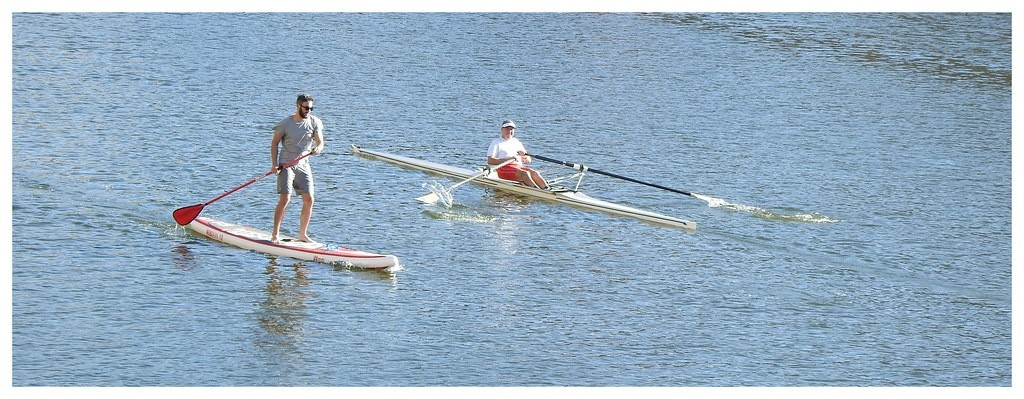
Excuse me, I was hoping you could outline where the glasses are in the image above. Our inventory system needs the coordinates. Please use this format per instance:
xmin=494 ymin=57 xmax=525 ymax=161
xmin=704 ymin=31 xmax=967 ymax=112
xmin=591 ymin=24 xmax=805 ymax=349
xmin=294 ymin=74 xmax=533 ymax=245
xmin=300 ymin=104 xmax=313 ymax=111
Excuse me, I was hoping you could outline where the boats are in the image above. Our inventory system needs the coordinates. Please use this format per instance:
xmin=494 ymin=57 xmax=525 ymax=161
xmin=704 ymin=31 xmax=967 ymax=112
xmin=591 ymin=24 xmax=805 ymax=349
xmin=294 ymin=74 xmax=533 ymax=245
xmin=349 ymin=142 xmax=697 ymax=235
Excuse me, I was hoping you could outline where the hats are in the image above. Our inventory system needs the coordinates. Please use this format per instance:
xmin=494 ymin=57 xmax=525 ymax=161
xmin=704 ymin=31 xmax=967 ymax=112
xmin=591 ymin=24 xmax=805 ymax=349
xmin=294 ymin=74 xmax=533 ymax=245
xmin=502 ymin=119 xmax=515 ymax=128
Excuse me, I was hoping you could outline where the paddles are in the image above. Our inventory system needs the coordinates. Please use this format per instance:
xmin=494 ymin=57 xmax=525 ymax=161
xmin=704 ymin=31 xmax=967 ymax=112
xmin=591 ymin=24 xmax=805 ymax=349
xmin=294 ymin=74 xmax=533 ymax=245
xmin=414 ymin=158 xmax=516 ymax=204
xmin=516 ymin=150 xmax=710 ymax=202
xmin=171 ymin=151 xmax=313 ymax=227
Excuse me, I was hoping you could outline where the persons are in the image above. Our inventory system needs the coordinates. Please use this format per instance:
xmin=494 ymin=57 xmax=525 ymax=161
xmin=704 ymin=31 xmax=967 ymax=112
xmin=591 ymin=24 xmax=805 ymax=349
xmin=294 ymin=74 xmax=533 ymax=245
xmin=487 ymin=120 xmax=550 ymax=191
xmin=270 ymin=93 xmax=324 ymax=243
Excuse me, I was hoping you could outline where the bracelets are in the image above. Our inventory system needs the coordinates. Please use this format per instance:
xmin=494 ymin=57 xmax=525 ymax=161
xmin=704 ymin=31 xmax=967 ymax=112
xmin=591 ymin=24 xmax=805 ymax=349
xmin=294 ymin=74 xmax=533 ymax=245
xmin=522 ymin=157 xmax=527 ymax=159
